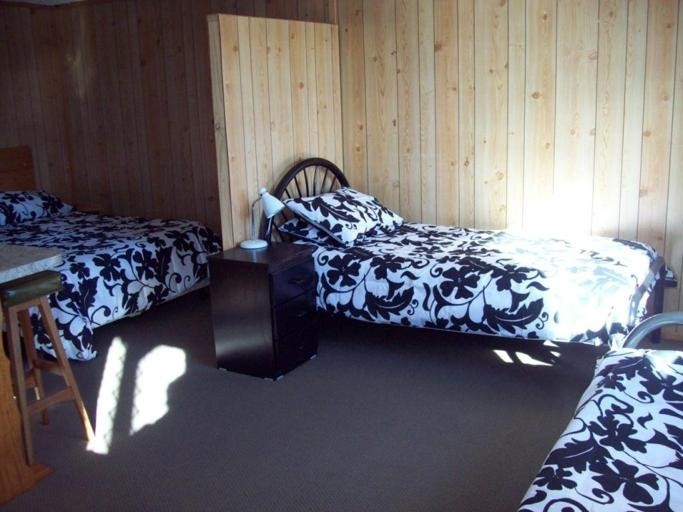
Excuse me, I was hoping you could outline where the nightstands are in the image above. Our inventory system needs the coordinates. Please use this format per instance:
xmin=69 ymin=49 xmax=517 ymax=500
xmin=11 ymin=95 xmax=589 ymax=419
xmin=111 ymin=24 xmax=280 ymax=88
xmin=206 ymin=241 xmax=320 ymax=380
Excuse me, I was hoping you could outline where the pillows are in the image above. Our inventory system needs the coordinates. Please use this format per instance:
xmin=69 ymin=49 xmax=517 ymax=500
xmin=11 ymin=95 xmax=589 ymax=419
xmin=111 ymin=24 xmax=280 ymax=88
xmin=279 ymin=186 xmax=403 ymax=248
xmin=0 ymin=190 xmax=74 ymax=225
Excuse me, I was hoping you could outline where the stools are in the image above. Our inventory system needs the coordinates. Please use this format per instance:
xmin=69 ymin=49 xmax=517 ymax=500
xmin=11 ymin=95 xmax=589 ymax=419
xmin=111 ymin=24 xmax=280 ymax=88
xmin=0 ymin=272 xmax=95 ymax=466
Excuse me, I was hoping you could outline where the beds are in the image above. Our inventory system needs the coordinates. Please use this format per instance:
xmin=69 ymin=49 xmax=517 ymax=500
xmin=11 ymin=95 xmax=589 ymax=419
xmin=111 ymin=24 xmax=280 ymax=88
xmin=0 ymin=146 xmax=221 ymax=357
xmin=518 ymin=312 xmax=683 ymax=512
xmin=263 ymin=158 xmax=662 ymax=347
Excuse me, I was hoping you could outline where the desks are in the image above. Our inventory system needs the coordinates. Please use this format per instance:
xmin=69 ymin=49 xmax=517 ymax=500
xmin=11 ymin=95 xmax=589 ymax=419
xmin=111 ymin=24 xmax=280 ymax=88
xmin=0 ymin=239 xmax=62 ymax=285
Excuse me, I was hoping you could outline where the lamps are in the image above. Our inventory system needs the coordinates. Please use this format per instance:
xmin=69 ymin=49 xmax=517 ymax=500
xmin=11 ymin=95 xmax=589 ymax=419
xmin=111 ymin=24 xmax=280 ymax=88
xmin=239 ymin=188 xmax=285 ymax=250
